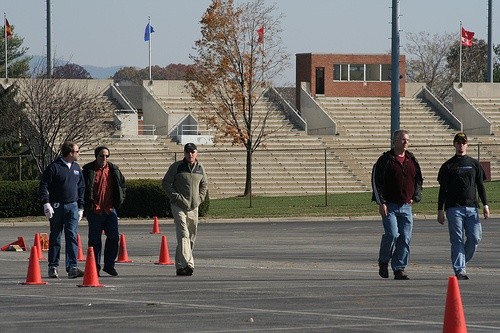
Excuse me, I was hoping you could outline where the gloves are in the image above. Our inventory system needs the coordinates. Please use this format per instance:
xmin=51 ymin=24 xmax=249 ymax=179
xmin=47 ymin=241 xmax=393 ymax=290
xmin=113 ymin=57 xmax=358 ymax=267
xmin=78 ymin=209 xmax=84 ymax=221
xmin=43 ymin=203 xmax=54 ymax=219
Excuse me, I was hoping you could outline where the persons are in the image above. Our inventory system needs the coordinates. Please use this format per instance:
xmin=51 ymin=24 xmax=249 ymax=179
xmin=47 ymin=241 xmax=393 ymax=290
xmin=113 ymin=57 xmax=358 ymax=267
xmin=82 ymin=147 xmax=127 ymax=276
xmin=437 ymin=133 xmax=489 ymax=280
xmin=371 ymin=130 xmax=424 ymax=280
xmin=162 ymin=142 xmax=208 ymax=276
xmin=38 ymin=142 xmax=86 ymax=279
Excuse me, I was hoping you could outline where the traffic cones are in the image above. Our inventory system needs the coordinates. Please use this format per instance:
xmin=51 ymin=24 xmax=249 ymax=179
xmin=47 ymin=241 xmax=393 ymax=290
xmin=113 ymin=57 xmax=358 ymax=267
xmin=28 ymin=232 xmax=45 ymax=259
xmin=154 ymin=235 xmax=174 ymax=265
xmin=75 ymin=246 xmax=103 ymax=287
xmin=19 ymin=247 xmax=47 ymax=284
xmin=0 ymin=237 xmax=27 ymax=251
xmin=150 ymin=216 xmax=162 ymax=234
xmin=443 ymin=276 xmax=467 ymax=333
xmin=115 ymin=233 xmax=132 ymax=263
xmin=77 ymin=235 xmax=86 ymax=261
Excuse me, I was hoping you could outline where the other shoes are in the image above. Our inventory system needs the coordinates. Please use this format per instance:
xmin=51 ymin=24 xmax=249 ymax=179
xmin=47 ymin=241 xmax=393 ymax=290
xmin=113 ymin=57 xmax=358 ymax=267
xmin=456 ymin=274 xmax=469 ymax=280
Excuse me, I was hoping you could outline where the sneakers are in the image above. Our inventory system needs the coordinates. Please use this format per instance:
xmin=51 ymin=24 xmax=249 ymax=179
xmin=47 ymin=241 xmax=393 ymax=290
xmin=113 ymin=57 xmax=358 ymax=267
xmin=48 ymin=266 xmax=58 ymax=277
xmin=97 ymin=265 xmax=101 ymax=276
xmin=68 ymin=266 xmax=85 ymax=278
xmin=184 ymin=265 xmax=194 ymax=276
xmin=103 ymin=267 xmax=118 ymax=275
xmin=394 ymin=271 xmax=408 ymax=279
xmin=379 ymin=261 xmax=388 ymax=278
xmin=176 ymin=267 xmax=184 ymax=276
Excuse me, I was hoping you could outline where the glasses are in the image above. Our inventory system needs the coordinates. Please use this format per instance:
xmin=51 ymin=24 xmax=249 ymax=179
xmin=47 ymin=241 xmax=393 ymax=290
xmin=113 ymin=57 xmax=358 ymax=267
xmin=185 ymin=150 xmax=196 ymax=153
xmin=71 ymin=150 xmax=79 ymax=153
xmin=98 ymin=154 xmax=109 ymax=158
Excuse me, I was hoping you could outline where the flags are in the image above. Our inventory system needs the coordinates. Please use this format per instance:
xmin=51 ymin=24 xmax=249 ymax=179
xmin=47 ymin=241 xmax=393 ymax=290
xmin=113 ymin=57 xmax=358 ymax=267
xmin=145 ymin=23 xmax=155 ymax=41
xmin=257 ymin=27 xmax=264 ymax=43
xmin=4 ymin=19 xmax=13 ymax=39
xmin=462 ymin=27 xmax=474 ymax=46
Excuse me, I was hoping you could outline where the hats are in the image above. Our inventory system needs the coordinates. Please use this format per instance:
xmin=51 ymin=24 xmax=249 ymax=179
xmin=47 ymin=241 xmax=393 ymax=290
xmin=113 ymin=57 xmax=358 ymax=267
xmin=184 ymin=143 xmax=197 ymax=150
xmin=454 ymin=132 xmax=468 ymax=142
